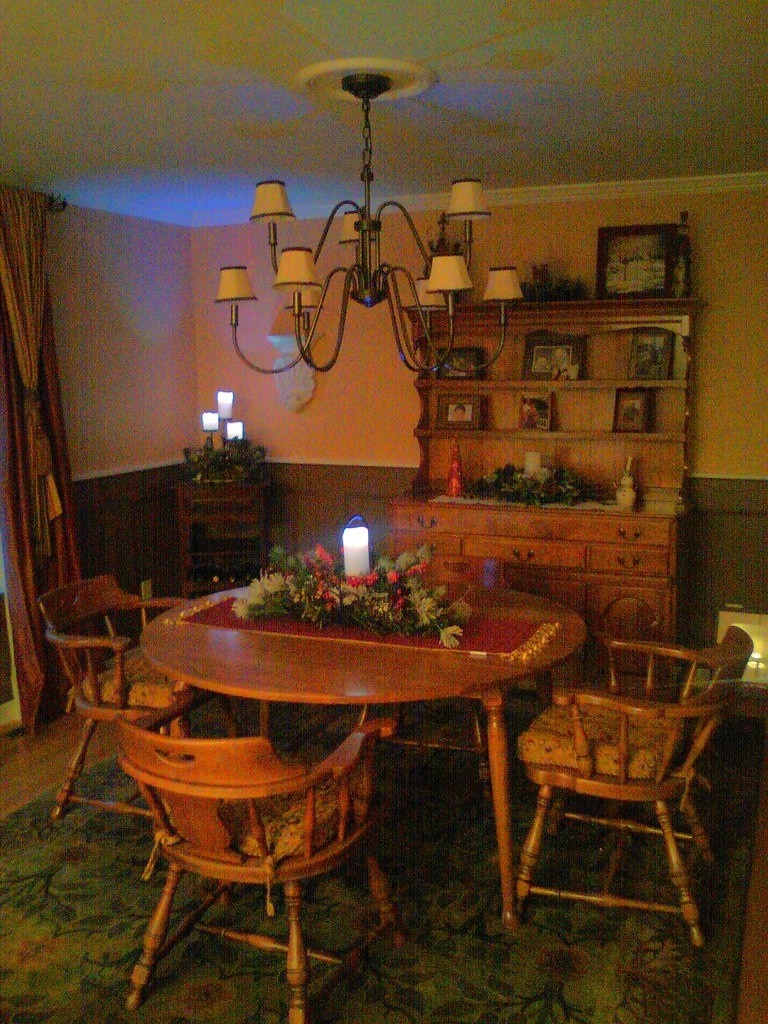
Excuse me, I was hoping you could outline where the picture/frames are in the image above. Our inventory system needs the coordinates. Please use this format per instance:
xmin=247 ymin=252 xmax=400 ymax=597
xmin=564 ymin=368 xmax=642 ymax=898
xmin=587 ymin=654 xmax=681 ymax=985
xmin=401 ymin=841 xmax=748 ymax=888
xmin=597 ymin=223 xmax=681 ymax=297
xmin=612 ymin=388 xmax=652 ymax=432
xmin=627 ymin=328 xmax=673 ymax=380
xmin=520 ymin=334 xmax=587 ymax=379
xmin=435 ymin=392 xmax=487 ymax=431
xmin=436 ymin=348 xmax=483 ymax=379
xmin=517 ymin=390 xmax=555 ymax=431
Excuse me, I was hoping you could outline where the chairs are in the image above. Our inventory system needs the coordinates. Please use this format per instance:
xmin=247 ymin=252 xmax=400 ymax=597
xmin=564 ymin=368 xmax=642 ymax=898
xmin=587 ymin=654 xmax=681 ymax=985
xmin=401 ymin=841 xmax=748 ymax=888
xmin=31 ymin=574 xmax=238 ymax=822
xmin=107 ymin=708 xmax=409 ymax=1023
xmin=517 ymin=623 xmax=755 ymax=949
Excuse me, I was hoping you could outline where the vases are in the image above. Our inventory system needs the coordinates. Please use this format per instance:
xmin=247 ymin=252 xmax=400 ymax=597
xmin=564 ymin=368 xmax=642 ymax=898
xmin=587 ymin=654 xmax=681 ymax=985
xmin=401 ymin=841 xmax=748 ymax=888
xmin=673 ymin=261 xmax=688 ymax=297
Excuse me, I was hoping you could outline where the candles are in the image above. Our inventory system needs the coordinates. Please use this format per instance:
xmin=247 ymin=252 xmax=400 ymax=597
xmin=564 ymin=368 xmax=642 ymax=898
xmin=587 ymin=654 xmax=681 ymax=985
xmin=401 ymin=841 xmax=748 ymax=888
xmin=226 ymin=422 xmax=243 ymax=439
xmin=202 ymin=411 xmax=218 ymax=430
xmin=626 ymin=455 xmax=633 ymax=470
xmin=526 ymin=449 xmax=541 ymax=474
xmin=342 ymin=526 xmax=370 ymax=576
xmin=217 ymin=388 xmax=233 ymax=419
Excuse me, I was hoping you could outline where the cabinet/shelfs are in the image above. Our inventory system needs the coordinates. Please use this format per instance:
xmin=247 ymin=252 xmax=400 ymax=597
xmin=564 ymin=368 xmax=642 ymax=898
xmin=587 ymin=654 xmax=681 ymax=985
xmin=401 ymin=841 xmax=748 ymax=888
xmin=177 ymin=483 xmax=269 ymax=594
xmin=389 ymin=295 xmax=710 ymax=672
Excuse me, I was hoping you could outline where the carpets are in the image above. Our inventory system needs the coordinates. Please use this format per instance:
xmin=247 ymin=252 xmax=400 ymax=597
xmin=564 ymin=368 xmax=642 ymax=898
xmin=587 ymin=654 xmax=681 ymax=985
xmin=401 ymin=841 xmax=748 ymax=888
xmin=0 ymin=702 xmax=765 ymax=1024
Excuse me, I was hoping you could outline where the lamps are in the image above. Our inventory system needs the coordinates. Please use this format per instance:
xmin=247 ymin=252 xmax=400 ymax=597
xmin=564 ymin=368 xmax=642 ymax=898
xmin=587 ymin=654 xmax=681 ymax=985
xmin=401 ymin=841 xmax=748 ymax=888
xmin=263 ymin=308 xmax=315 ymax=413
xmin=213 ymin=58 xmax=528 ymax=374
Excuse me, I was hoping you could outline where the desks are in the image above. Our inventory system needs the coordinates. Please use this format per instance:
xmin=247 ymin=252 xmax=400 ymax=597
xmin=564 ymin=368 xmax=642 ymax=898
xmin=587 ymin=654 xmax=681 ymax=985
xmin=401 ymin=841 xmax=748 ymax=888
xmin=138 ymin=584 xmax=590 ymax=929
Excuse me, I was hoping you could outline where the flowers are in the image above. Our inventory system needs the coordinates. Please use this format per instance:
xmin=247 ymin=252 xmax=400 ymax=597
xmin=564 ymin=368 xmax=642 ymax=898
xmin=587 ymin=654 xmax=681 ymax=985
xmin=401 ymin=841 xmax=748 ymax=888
xmin=519 ymin=262 xmax=592 ymax=301
xmin=472 ymin=462 xmax=614 ymax=506
xmin=234 ymin=545 xmax=475 ymax=648
xmin=677 ymin=209 xmax=691 ymax=258
